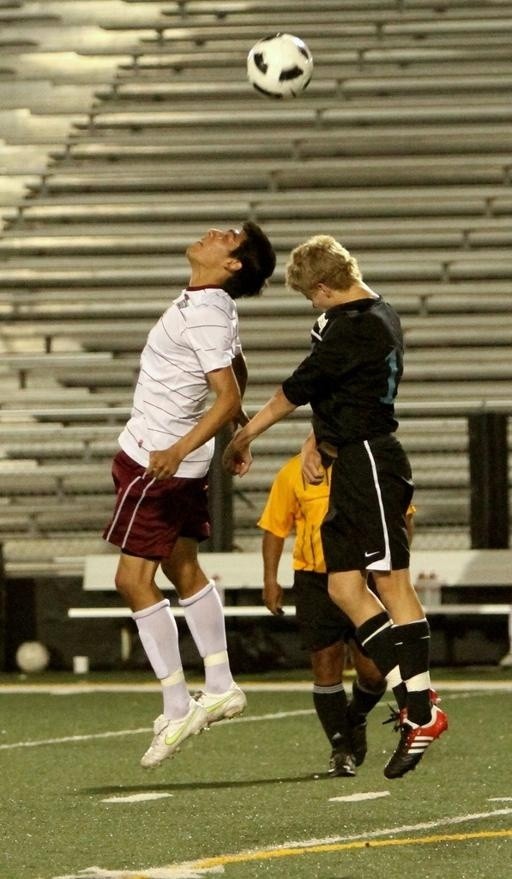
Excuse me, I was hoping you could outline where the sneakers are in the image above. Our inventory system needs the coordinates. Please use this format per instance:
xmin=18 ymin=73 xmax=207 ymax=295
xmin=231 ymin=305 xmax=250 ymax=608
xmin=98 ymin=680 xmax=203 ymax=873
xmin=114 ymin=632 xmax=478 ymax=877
xmin=328 ymin=719 xmax=367 ymax=776
xmin=140 ymin=682 xmax=248 ymax=766
xmin=382 ymin=690 xmax=448 ymax=778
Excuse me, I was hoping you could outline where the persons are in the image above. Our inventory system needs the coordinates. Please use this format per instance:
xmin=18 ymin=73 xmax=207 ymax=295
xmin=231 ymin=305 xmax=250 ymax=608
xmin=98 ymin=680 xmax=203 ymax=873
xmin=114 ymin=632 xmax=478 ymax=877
xmin=102 ymin=222 xmax=276 ymax=770
xmin=257 ymin=453 xmax=416 ymax=776
xmin=222 ymin=235 xmax=448 ymax=779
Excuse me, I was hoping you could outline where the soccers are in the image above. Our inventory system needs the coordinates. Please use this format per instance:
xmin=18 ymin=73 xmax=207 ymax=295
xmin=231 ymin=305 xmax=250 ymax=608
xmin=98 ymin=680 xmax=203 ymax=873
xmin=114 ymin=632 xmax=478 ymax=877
xmin=247 ymin=32 xmax=312 ymax=101
xmin=16 ymin=642 xmax=49 ymax=671
